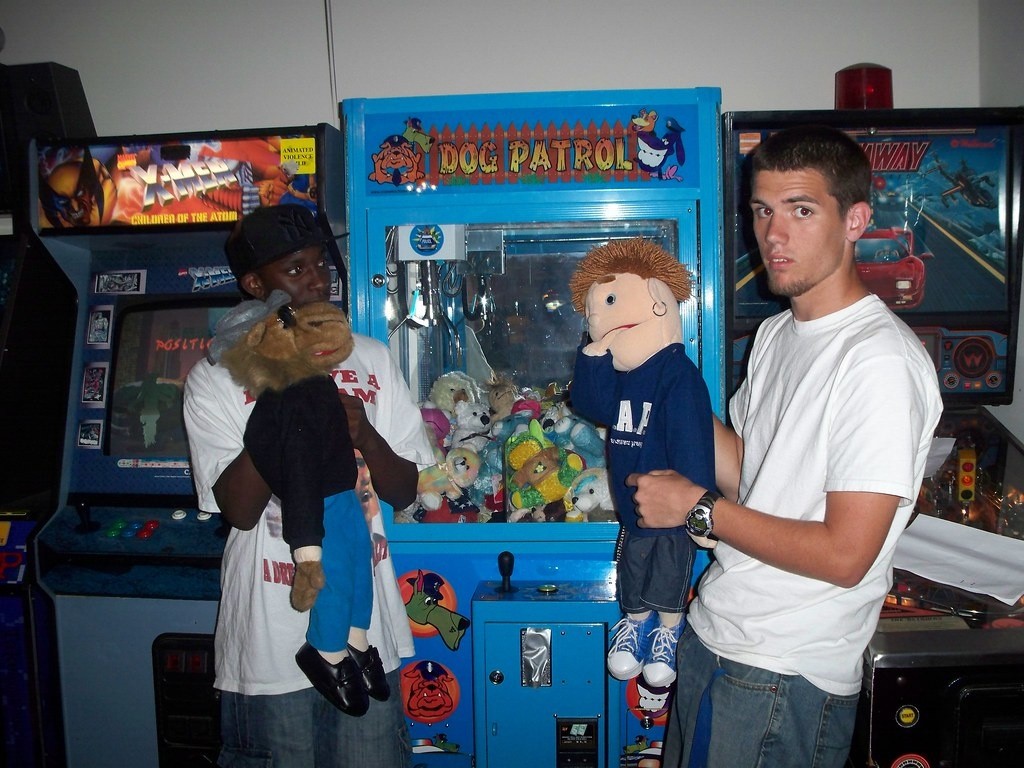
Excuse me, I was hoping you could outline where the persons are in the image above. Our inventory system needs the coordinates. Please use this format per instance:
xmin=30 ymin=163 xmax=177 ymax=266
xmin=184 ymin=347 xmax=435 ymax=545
xmin=183 ymin=205 xmax=437 ymax=768
xmin=568 ymin=235 xmax=717 ymax=688
xmin=625 ymin=123 xmax=944 ymax=768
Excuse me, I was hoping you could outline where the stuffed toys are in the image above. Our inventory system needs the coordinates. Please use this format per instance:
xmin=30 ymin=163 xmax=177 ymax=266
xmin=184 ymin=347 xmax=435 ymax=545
xmin=209 ymin=289 xmax=391 ymax=717
xmin=393 ymin=370 xmax=619 ymax=523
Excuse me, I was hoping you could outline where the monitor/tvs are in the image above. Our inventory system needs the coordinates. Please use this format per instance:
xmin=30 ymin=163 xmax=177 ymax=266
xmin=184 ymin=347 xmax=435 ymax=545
xmin=103 ymin=305 xmax=236 ymax=457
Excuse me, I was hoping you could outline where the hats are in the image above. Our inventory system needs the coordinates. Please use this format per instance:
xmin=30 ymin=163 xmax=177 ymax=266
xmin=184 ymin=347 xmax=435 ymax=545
xmin=223 ymin=202 xmax=350 ymax=280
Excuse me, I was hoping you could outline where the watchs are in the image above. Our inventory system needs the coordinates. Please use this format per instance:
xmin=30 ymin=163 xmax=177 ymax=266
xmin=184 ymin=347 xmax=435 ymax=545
xmin=685 ymin=490 xmax=725 ymax=537
